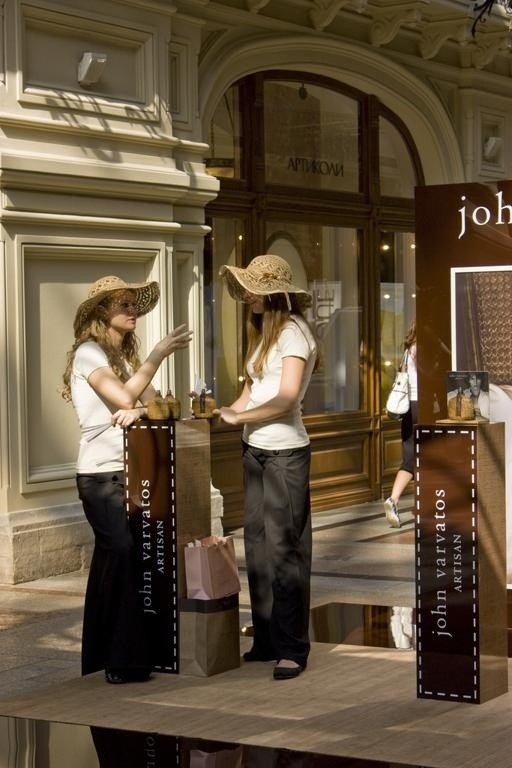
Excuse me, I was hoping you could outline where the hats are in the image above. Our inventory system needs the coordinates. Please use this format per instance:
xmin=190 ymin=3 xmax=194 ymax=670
xmin=74 ymin=274 xmax=161 ymax=338
xmin=219 ymin=254 xmax=312 ymax=312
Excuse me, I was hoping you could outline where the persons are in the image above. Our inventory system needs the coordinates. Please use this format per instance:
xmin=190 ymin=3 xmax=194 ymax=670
xmin=60 ymin=271 xmax=196 ymax=687
xmin=387 ymin=606 xmax=414 ymax=650
xmin=89 ymin=724 xmax=189 ymax=768
xmin=384 ymin=322 xmax=418 ymax=528
xmin=210 ymin=251 xmax=321 ymax=682
xmin=446 ymin=372 xmax=489 ymax=422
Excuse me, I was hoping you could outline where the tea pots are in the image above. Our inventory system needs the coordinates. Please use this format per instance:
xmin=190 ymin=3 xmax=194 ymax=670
xmin=165 ymin=390 xmax=181 ymax=420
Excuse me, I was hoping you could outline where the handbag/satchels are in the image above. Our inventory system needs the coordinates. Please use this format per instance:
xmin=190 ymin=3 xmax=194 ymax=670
xmin=178 ymin=535 xmax=242 ymax=677
xmin=385 ymin=372 xmax=411 ymax=420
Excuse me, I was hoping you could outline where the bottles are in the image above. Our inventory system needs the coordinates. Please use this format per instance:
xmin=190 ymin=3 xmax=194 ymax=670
xmin=147 ymin=391 xmax=170 ymax=421
xmin=448 ymin=386 xmax=475 ymax=420
xmin=192 ymin=388 xmax=217 ymax=418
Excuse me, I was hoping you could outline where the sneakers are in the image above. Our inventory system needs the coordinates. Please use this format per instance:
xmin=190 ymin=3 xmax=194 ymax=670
xmin=383 ymin=497 xmax=401 ymax=528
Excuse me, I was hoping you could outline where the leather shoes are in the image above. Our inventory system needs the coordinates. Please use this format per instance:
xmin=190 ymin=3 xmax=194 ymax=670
xmin=273 ymin=665 xmax=302 ymax=680
xmin=243 ymin=652 xmax=277 ymax=661
